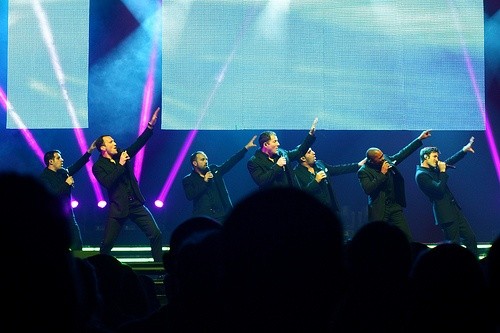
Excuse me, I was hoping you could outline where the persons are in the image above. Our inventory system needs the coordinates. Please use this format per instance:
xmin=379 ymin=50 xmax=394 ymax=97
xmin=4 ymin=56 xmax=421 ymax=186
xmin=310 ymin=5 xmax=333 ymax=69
xmin=294 ymin=144 xmax=367 ymax=216
xmin=357 ymin=128 xmax=434 ymax=243
xmin=92 ymin=106 xmax=164 ymax=263
xmin=247 ymin=114 xmax=319 ymax=191
xmin=41 ymin=137 xmax=98 ymax=252
xmin=0 ymin=172 xmax=500 ymax=332
xmin=415 ymin=135 xmax=477 ymax=258
xmin=183 ymin=134 xmax=258 ymax=225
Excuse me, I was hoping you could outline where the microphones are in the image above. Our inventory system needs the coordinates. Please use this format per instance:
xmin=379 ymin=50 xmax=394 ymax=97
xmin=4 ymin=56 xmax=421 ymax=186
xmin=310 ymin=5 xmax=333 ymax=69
xmin=436 ymin=162 xmax=456 ymax=169
xmin=64 ymin=169 xmax=75 ymax=188
xmin=118 ymin=147 xmax=131 ymax=162
xmin=279 ymin=151 xmax=285 ymax=172
xmin=206 ymin=166 xmax=214 ymax=183
xmin=318 ymin=167 xmax=328 ymax=184
xmin=382 ymin=161 xmax=395 ymax=175
